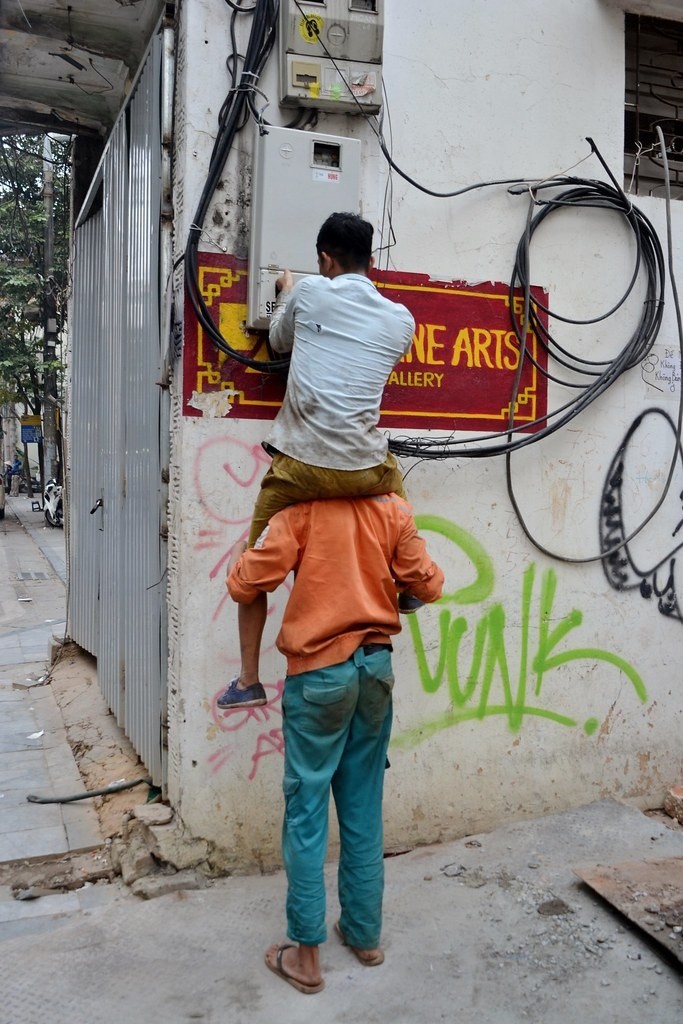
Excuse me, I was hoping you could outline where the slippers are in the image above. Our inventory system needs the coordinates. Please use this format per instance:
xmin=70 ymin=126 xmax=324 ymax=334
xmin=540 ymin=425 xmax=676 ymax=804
xmin=265 ymin=944 xmax=325 ymax=994
xmin=334 ymin=921 xmax=385 ymax=966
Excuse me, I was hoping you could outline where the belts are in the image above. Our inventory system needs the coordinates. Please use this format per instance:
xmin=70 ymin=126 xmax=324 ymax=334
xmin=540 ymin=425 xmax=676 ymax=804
xmin=350 ymin=643 xmax=387 ymax=659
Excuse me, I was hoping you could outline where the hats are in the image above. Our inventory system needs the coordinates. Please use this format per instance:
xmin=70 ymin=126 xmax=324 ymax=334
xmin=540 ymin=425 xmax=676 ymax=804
xmin=4 ymin=461 xmax=12 ymax=467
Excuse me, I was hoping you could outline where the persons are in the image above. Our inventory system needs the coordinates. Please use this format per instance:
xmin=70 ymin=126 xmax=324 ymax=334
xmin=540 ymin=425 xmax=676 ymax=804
xmin=3 ymin=455 xmax=22 ymax=496
xmin=226 ymin=493 xmax=445 ymax=993
xmin=215 ymin=211 xmax=429 ymax=708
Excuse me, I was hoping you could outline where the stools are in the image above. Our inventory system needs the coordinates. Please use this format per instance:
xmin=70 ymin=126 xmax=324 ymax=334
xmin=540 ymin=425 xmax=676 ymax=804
xmin=31 ymin=501 xmax=41 ymax=511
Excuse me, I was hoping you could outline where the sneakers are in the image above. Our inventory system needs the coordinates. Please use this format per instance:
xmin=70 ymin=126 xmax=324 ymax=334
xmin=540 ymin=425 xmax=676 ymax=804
xmin=217 ymin=677 xmax=266 ymax=708
xmin=397 ymin=593 xmax=424 ymax=613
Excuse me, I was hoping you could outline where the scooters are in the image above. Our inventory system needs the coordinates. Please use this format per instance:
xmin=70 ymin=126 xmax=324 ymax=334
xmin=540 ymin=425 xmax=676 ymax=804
xmin=42 ymin=478 xmax=63 ymax=528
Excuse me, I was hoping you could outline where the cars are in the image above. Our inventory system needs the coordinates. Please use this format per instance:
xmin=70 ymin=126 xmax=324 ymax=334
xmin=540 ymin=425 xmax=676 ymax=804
xmin=19 ymin=476 xmax=41 ymax=493
